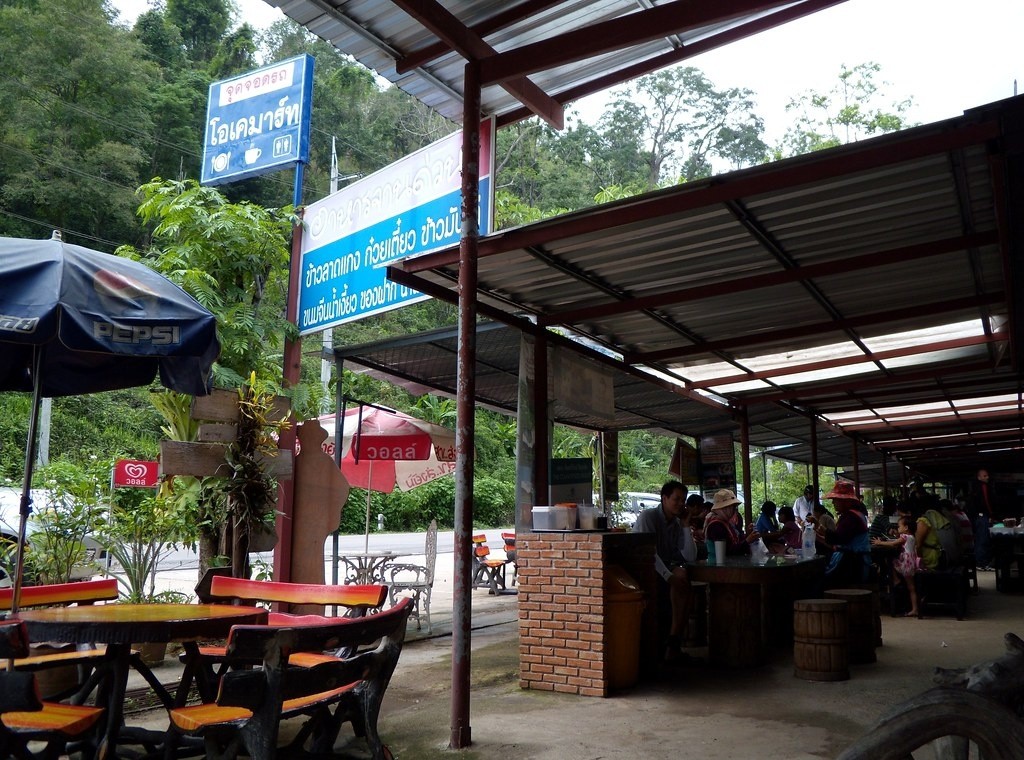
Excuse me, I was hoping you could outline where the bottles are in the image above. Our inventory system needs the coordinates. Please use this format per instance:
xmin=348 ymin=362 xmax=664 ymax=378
xmin=802 ymin=526 xmax=816 ymax=558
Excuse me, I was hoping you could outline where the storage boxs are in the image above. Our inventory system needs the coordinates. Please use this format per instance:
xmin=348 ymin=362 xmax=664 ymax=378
xmin=531 ymin=506 xmax=567 ymax=530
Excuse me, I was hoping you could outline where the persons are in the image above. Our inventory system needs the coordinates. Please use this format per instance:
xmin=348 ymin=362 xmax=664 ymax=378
xmin=631 ymin=468 xmax=999 ymax=662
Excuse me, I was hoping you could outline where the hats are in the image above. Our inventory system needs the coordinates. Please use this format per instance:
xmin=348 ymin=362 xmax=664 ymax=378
xmin=687 ymin=495 xmax=704 ymax=505
xmin=825 ymin=480 xmax=859 ymax=500
xmin=804 ymin=485 xmax=813 ymax=494
xmin=711 ymin=489 xmax=741 ymax=509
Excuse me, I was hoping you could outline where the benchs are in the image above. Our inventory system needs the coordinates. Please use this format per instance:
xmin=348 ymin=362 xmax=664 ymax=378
xmin=0 ymin=576 xmax=413 ymax=760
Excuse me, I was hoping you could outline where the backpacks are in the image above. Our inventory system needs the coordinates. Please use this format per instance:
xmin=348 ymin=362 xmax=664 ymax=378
xmin=922 ymin=514 xmax=960 ymax=569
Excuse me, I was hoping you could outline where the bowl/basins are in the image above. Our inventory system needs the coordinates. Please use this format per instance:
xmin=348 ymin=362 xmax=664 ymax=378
xmin=783 ymin=555 xmax=797 ymax=562
xmin=887 ymin=539 xmax=898 ymax=542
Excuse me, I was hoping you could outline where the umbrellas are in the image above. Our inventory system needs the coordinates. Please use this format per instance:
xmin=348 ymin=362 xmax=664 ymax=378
xmin=0 ymin=230 xmax=221 ymax=612
xmin=262 ymin=402 xmax=478 ymax=553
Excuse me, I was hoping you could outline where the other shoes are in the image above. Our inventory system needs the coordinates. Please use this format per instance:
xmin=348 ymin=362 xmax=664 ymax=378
xmin=904 ymin=612 xmax=919 ymax=617
xmin=984 ymin=562 xmax=996 ymax=571
xmin=976 ymin=566 xmax=985 ymax=572
xmin=921 ymin=595 xmax=928 ymax=610
xmin=893 ymin=579 xmax=902 ymax=588
xmin=664 ymin=634 xmax=681 ymax=652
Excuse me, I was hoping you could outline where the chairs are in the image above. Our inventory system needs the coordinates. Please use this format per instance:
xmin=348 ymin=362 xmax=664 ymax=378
xmin=473 ymin=533 xmax=517 ymax=594
xmin=379 ymin=519 xmax=438 ymax=635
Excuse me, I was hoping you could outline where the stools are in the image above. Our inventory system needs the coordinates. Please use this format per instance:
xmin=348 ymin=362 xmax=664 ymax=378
xmin=919 ymin=550 xmax=981 ymax=620
xmin=821 ymin=583 xmax=887 ymax=663
xmin=795 ymin=599 xmax=850 ymax=680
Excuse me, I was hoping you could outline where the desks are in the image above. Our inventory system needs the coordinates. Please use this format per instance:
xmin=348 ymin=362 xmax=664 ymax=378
xmin=323 ymin=549 xmax=413 ymax=619
xmin=17 ymin=607 xmax=268 ymax=760
xmin=701 ymin=550 xmax=835 ymax=672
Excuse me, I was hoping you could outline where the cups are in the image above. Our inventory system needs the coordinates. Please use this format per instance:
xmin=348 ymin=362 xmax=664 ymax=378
xmin=714 ymin=537 xmax=727 ymax=564
xmin=794 ymin=548 xmax=802 ymax=555
xmin=579 ymin=504 xmax=598 ymax=529
